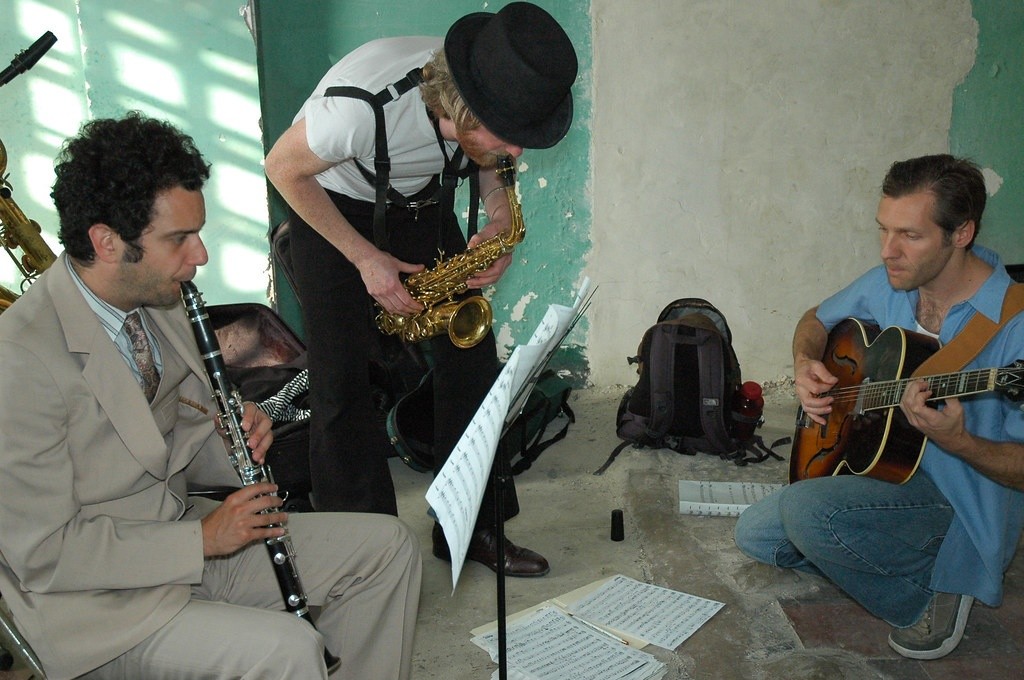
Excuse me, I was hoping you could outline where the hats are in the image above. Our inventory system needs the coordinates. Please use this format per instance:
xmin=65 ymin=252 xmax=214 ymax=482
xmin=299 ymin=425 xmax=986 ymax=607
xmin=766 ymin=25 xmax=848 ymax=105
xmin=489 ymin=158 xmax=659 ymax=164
xmin=443 ymin=1 xmax=578 ymax=149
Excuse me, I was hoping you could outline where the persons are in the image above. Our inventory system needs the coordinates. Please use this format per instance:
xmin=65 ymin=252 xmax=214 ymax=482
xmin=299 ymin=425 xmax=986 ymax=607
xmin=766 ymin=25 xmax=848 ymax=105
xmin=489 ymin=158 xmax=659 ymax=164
xmin=0 ymin=110 xmax=423 ymax=679
xmin=263 ymin=2 xmax=578 ymax=577
xmin=734 ymin=154 xmax=1024 ymax=660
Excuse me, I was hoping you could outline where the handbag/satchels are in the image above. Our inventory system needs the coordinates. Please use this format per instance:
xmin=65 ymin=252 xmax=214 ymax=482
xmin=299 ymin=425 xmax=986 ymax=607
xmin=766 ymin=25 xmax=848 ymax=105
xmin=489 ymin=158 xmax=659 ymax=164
xmin=186 ymin=302 xmax=312 ymax=505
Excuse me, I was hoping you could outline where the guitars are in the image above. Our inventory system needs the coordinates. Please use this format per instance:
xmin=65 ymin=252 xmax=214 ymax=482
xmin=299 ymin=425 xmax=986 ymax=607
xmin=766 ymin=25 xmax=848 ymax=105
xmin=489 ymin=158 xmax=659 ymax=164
xmin=787 ymin=316 xmax=1024 ymax=485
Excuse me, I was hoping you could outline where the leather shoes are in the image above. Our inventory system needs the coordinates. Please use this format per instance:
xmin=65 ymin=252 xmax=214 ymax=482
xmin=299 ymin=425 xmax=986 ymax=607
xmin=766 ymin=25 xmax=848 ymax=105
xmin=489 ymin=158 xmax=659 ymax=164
xmin=432 ymin=523 xmax=550 ymax=578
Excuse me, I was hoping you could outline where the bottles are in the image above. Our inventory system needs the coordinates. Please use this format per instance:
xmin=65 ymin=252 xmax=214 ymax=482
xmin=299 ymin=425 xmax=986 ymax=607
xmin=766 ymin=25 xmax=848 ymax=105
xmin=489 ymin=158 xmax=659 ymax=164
xmin=729 ymin=380 xmax=765 ymax=439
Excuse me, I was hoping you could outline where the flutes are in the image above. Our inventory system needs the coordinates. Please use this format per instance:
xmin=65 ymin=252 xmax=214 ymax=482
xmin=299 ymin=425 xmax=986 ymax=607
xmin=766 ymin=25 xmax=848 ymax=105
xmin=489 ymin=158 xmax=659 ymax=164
xmin=181 ymin=278 xmax=342 ymax=676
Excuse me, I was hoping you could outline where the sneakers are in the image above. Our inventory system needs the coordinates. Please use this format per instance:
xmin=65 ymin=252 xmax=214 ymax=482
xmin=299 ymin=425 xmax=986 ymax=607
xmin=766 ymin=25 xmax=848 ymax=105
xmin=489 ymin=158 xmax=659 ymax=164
xmin=887 ymin=591 xmax=974 ymax=659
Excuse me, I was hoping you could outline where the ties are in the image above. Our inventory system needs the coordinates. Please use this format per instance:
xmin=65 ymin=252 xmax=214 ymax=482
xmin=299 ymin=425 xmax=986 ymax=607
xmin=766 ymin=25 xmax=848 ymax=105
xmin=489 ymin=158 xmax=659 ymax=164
xmin=124 ymin=311 xmax=160 ymax=404
xmin=425 ymin=104 xmax=464 ymax=251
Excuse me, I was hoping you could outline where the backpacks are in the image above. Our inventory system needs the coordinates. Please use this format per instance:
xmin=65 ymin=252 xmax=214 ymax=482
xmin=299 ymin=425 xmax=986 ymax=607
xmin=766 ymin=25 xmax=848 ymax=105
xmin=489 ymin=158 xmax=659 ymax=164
xmin=592 ymin=298 xmax=792 ymax=477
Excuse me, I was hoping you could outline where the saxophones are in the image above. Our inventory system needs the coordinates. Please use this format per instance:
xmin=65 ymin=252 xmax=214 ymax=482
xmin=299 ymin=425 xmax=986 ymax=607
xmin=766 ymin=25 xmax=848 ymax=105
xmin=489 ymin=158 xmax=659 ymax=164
xmin=374 ymin=155 xmax=527 ymax=349
xmin=0 ymin=31 xmax=58 ymax=318
